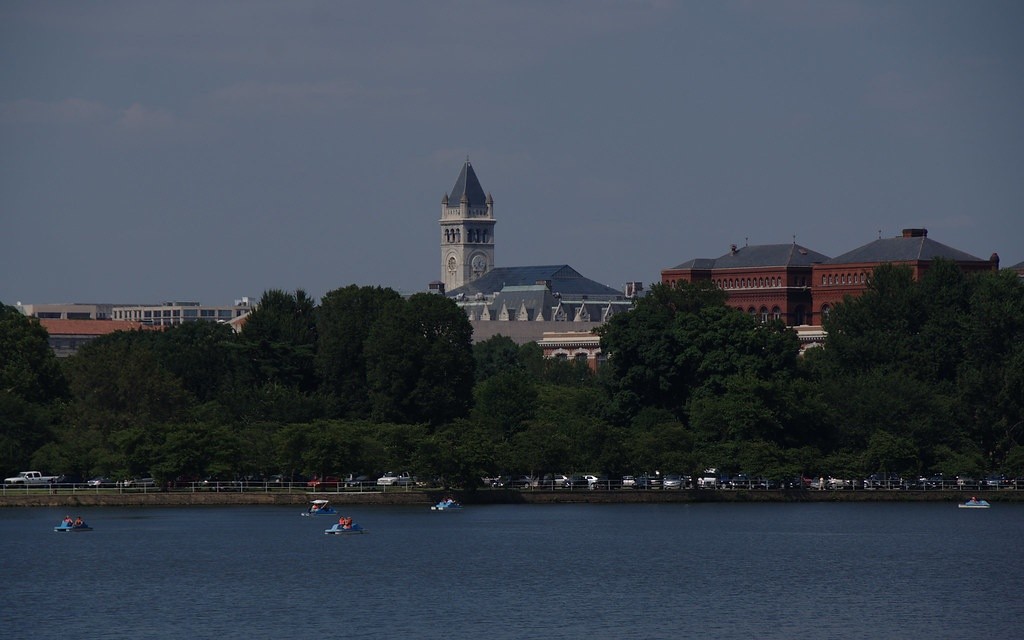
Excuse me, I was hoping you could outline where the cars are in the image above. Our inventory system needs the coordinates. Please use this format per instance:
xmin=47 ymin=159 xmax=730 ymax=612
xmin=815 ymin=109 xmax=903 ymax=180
xmin=919 ymin=473 xmax=949 ymax=487
xmin=493 ymin=475 xmax=533 ymax=489
xmin=248 ymin=473 xmax=370 ymax=491
xmin=87 ymin=476 xmax=116 ymax=488
xmin=561 ymin=476 xmax=588 ymax=488
xmin=538 ymin=475 xmax=570 ymax=485
xmin=198 ymin=482 xmax=225 ymax=492
xmin=116 ymin=478 xmax=157 ymax=488
xmin=664 ymin=475 xmax=686 ymax=489
xmin=582 ymin=475 xmax=599 ymax=486
xmin=824 ymin=475 xmax=870 ymax=490
xmin=623 ymin=476 xmax=637 ymax=486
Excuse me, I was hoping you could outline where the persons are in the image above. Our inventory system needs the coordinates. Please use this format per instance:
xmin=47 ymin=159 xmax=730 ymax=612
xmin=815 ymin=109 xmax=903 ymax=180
xmin=337 ymin=516 xmax=352 ymax=529
xmin=312 ymin=503 xmax=328 ymax=511
xmin=72 ymin=517 xmax=83 ymax=526
xmin=969 ymin=496 xmax=979 ymax=504
xmin=64 ymin=514 xmax=73 ymax=523
xmin=441 ymin=497 xmax=459 ymax=506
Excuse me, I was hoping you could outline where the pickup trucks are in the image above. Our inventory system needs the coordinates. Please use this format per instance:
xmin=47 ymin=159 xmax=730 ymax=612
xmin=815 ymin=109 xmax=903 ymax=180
xmin=377 ymin=471 xmax=417 ymax=486
xmin=4 ymin=471 xmax=59 ymax=488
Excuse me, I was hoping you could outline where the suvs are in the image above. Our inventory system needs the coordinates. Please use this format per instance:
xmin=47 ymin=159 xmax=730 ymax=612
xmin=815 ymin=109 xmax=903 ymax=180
xmin=590 ymin=476 xmax=624 ymax=490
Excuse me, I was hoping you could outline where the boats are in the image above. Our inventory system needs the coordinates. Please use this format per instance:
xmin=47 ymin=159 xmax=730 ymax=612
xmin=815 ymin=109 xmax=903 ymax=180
xmin=53 ymin=522 xmax=94 ymax=532
xmin=431 ymin=501 xmax=464 ymax=513
xmin=301 ymin=500 xmax=340 ymax=517
xmin=958 ymin=496 xmax=991 ymax=509
xmin=325 ymin=523 xmax=369 ymax=535
